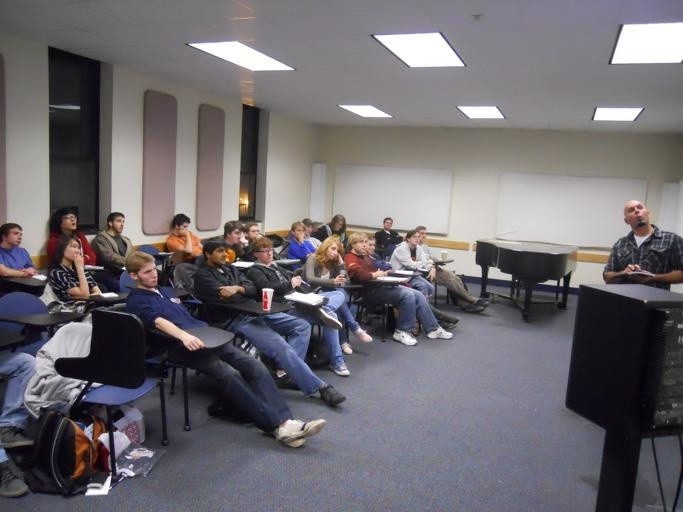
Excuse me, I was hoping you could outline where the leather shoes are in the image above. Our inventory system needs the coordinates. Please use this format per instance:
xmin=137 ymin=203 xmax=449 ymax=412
xmin=355 ymin=328 xmax=373 ymax=343
xmin=342 ymin=343 xmax=352 ymax=355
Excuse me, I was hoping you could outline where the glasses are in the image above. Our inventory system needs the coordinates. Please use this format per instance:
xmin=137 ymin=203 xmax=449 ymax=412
xmin=254 ymin=249 xmax=273 ymax=253
xmin=61 ymin=215 xmax=76 ymax=219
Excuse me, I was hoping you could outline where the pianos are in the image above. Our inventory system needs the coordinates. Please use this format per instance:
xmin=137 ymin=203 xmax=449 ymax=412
xmin=475 ymin=237 xmax=578 ymax=321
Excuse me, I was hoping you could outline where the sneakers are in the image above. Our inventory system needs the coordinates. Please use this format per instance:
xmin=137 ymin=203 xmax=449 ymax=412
xmin=315 ymin=305 xmax=343 ymax=331
xmin=277 ymin=418 xmax=328 ymax=441
xmin=464 ymin=303 xmax=485 ymax=312
xmin=273 ymin=373 xmax=299 ymax=389
xmin=393 ymin=330 xmax=418 ymax=346
xmin=0 ymin=427 xmax=34 ymax=448
xmin=426 ymin=327 xmax=453 ymax=339
xmin=0 ymin=461 xmax=28 ymax=498
xmin=319 ymin=382 xmax=346 ymax=405
xmin=329 ymin=361 xmax=349 ymax=376
xmin=475 ymin=296 xmax=490 ymax=306
xmin=272 ymin=429 xmax=306 ymax=447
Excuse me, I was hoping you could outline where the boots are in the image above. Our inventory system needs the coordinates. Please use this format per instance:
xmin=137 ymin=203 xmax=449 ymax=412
xmin=430 ymin=304 xmax=461 ymax=323
xmin=441 ymin=321 xmax=458 ymax=329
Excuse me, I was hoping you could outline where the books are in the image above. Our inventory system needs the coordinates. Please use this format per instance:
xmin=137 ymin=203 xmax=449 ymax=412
xmin=617 ymin=271 xmax=656 ymax=284
xmin=284 ymin=292 xmax=324 ymax=306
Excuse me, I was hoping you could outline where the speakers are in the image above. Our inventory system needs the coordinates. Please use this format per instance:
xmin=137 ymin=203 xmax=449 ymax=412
xmin=564 ymin=282 xmax=682 ymax=439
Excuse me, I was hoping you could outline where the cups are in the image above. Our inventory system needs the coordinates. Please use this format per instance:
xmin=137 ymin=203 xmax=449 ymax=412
xmin=440 ymin=251 xmax=448 ymax=261
xmin=261 ymin=287 xmax=274 ymax=313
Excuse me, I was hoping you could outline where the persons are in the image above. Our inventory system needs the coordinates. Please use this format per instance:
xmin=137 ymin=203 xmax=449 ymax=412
xmin=126 ymin=251 xmax=326 ymax=448
xmin=603 ymin=201 xmax=682 ymax=292
xmin=0 ymin=350 xmax=35 ymax=498
xmin=2 ymin=205 xmax=491 ymax=407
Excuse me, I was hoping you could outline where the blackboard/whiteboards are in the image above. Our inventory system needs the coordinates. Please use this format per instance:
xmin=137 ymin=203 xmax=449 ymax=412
xmin=332 ymin=163 xmax=452 ymax=236
xmin=495 ymin=171 xmax=651 ymax=251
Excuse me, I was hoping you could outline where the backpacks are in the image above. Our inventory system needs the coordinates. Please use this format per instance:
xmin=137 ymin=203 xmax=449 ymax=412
xmin=25 ymin=408 xmax=95 ymax=495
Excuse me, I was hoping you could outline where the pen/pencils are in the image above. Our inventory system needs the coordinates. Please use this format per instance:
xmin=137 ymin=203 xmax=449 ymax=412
xmin=291 ymin=271 xmax=310 ymax=287
